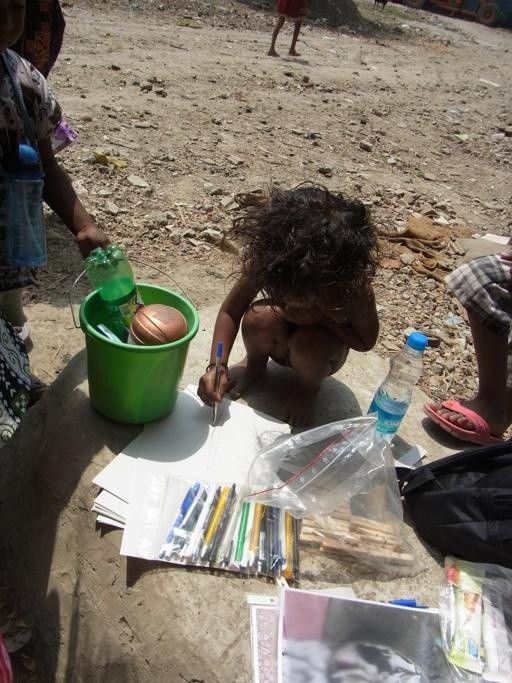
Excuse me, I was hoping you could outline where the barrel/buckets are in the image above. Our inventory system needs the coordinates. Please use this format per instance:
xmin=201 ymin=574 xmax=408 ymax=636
xmin=69 ymin=256 xmax=199 ymax=424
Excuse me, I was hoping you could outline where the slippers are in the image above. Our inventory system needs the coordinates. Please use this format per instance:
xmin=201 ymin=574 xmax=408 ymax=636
xmin=422 ymin=399 xmax=505 ymax=446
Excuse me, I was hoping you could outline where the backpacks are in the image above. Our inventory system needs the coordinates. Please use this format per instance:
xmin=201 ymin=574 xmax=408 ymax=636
xmin=395 ymin=438 xmax=512 ymax=569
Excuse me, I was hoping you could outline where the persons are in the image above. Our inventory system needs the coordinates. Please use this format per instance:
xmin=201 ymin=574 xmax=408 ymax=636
xmin=195 ymin=179 xmax=380 ymax=430
xmin=420 ymin=244 xmax=512 ymax=447
xmin=1 ymin=0 xmax=109 ymax=453
xmin=265 ymin=0 xmax=305 ymax=58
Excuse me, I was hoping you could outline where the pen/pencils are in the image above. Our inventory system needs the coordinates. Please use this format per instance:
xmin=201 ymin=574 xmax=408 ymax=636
xmin=159 ymin=480 xmax=299 ymax=582
xmin=212 ymin=342 xmax=222 ymax=427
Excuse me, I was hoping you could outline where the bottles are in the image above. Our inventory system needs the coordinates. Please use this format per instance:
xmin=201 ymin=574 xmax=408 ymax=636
xmin=7 ymin=143 xmax=47 ymax=269
xmin=365 ymin=332 xmax=430 ymax=442
xmin=85 ymin=243 xmax=146 ymax=341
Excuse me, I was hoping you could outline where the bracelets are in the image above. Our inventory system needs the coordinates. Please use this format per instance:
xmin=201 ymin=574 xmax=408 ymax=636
xmin=206 ymin=362 xmax=228 ymax=372
